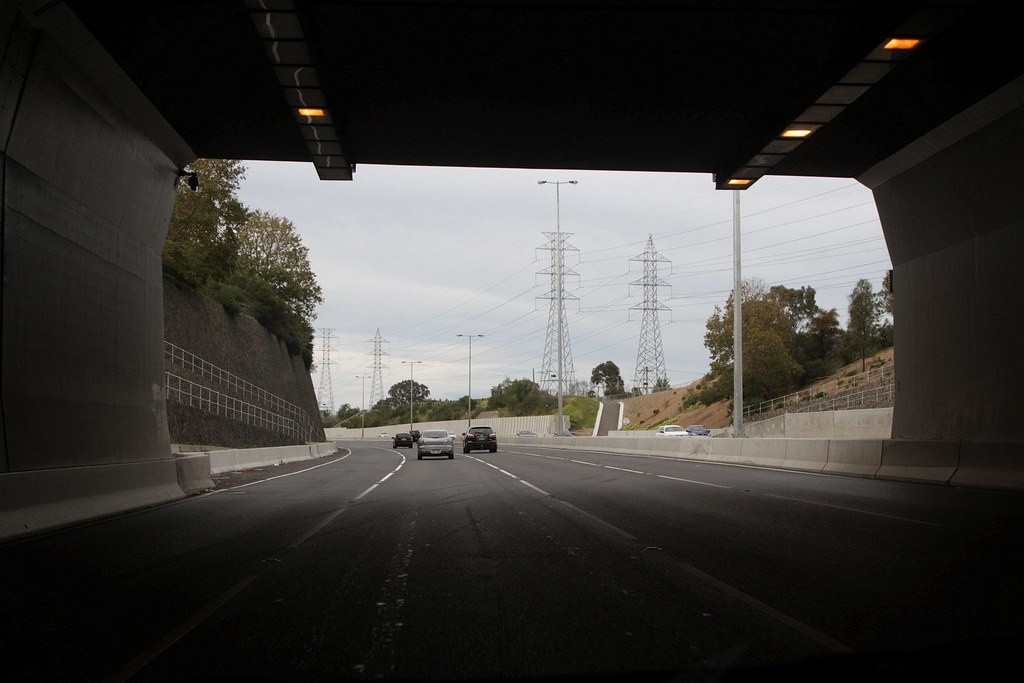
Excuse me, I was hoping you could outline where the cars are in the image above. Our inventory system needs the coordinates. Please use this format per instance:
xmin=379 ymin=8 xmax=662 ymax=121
xmin=655 ymin=425 xmax=688 ymax=437
xmin=516 ymin=431 xmax=536 ymax=437
xmin=392 ymin=433 xmax=413 ymax=448
xmin=553 ymin=428 xmax=574 ymax=436
xmin=377 ymin=433 xmax=388 ymax=437
xmin=685 ymin=425 xmax=711 ymax=437
xmin=449 ymin=432 xmax=456 ymax=440
xmin=416 ymin=431 xmax=455 ymax=459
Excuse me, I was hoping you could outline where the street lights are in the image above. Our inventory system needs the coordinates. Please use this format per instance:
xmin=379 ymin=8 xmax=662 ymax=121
xmin=356 ymin=376 xmax=372 ymax=438
xmin=538 ymin=179 xmax=580 ymax=437
xmin=456 ymin=334 xmax=484 ymax=428
xmin=402 ymin=361 xmax=422 ymax=431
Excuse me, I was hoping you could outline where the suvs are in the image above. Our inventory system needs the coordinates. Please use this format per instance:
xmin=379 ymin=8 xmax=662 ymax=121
xmin=462 ymin=426 xmax=497 ymax=453
xmin=410 ymin=431 xmax=420 ymax=442
